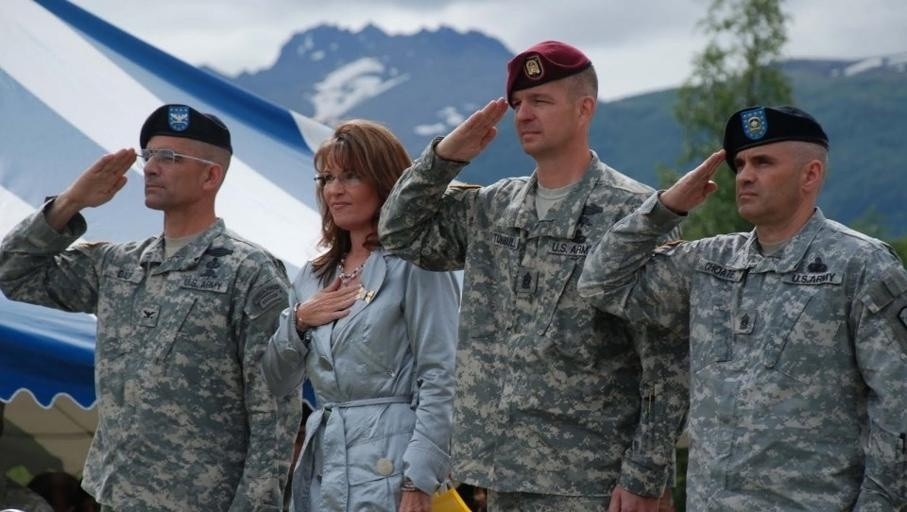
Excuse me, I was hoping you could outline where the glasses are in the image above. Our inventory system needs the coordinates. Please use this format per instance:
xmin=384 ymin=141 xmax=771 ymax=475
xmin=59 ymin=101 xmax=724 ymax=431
xmin=140 ymin=149 xmax=213 ymax=165
xmin=313 ymin=174 xmax=360 ymax=188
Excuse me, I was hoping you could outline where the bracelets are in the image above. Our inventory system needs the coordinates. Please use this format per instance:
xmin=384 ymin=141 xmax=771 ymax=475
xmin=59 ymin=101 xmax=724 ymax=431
xmin=400 ymin=481 xmax=416 ymax=491
xmin=294 ymin=302 xmax=310 ymax=328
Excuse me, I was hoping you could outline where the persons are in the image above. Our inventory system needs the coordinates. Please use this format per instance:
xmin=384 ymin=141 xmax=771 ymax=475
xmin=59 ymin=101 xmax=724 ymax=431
xmin=0 ymin=104 xmax=302 ymax=512
xmin=577 ymin=106 xmax=907 ymax=512
xmin=263 ymin=119 xmax=460 ymax=512
xmin=377 ymin=40 xmax=691 ymax=512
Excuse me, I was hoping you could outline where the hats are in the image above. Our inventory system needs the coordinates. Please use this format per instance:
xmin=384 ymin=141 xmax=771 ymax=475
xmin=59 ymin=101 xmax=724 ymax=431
xmin=140 ymin=103 xmax=233 ymax=155
xmin=507 ymin=41 xmax=592 ymax=108
xmin=723 ymin=105 xmax=829 ymax=173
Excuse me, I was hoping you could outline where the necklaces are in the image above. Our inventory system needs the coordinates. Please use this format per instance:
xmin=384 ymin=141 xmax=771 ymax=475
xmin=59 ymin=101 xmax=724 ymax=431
xmin=339 ymin=252 xmax=364 ymax=279
